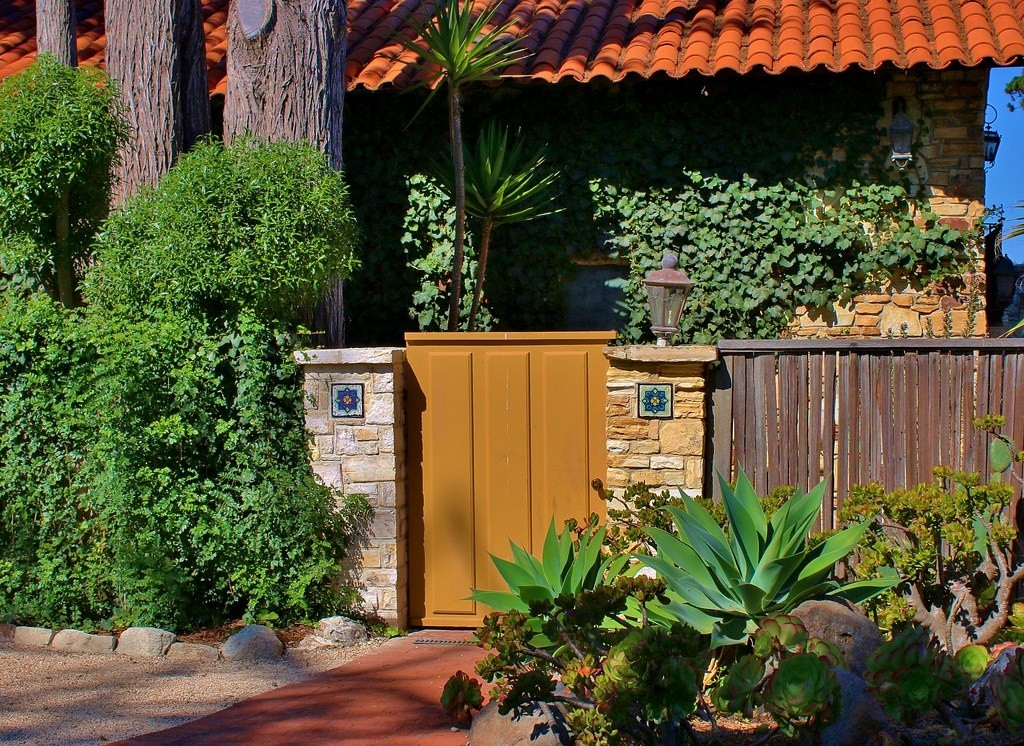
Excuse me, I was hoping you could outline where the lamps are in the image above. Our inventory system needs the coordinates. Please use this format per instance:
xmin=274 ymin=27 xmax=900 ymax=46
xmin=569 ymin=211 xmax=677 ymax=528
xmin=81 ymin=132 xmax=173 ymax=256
xmin=888 ymin=96 xmax=916 ymax=168
xmin=641 ymin=255 xmax=696 ymax=346
xmin=982 ymin=104 xmax=1002 ymax=174
